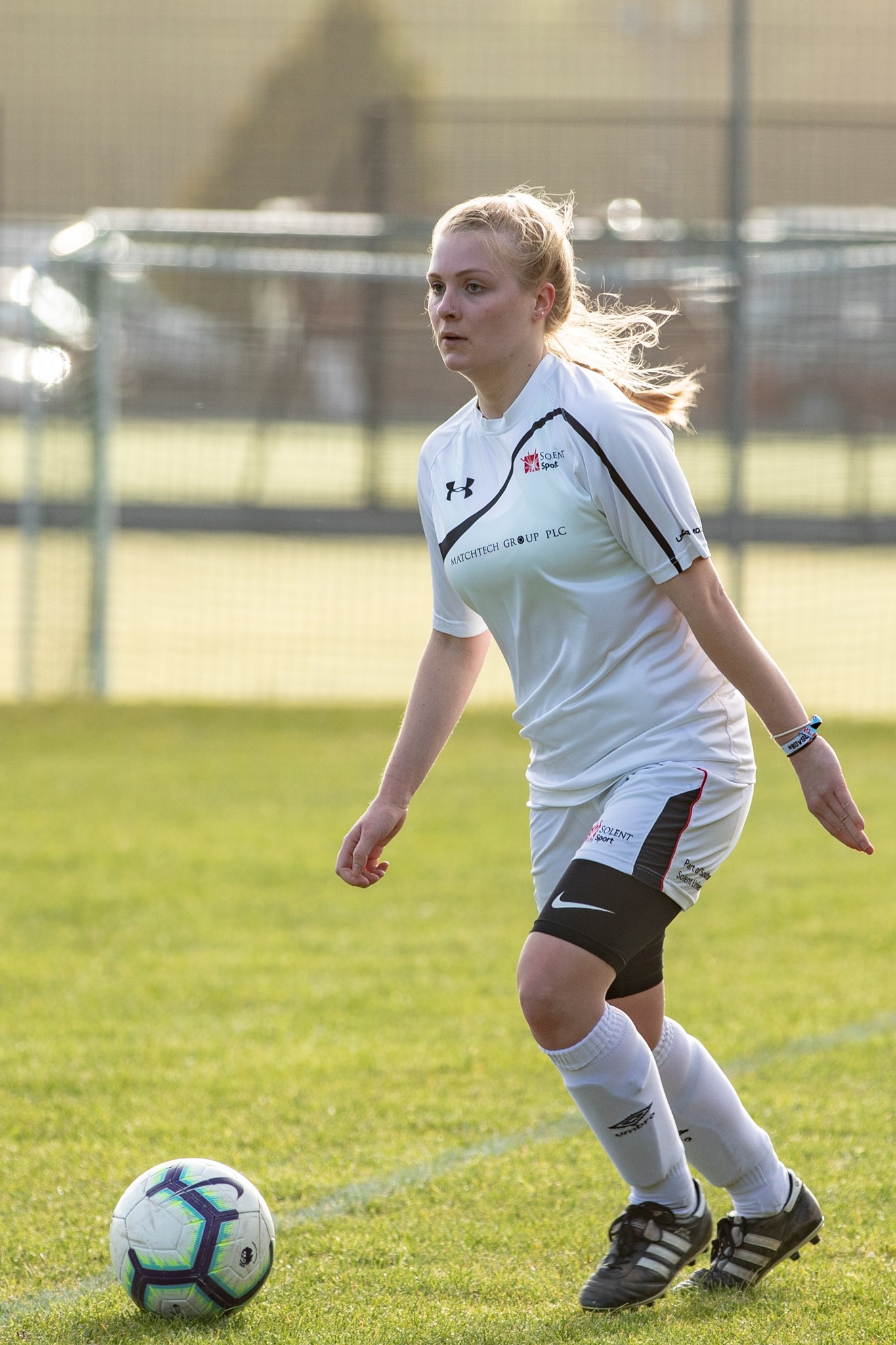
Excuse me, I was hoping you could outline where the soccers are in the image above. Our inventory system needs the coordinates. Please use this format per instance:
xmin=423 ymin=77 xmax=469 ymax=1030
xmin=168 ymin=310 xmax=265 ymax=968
xmin=109 ymin=1159 xmax=276 ymax=1319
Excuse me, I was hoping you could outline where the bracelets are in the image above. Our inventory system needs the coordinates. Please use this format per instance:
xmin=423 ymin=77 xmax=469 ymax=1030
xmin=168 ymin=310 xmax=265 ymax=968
xmin=779 ymin=715 xmax=823 ymax=757
xmin=771 ymin=720 xmax=811 ymax=738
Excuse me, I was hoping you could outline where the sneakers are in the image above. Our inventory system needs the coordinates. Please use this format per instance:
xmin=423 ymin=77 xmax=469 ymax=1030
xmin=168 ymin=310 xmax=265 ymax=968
xmin=578 ymin=1176 xmax=713 ymax=1315
xmin=672 ymin=1168 xmax=825 ymax=1301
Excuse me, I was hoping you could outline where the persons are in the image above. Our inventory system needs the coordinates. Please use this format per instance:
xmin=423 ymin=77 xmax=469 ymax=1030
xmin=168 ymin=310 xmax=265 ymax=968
xmin=337 ymin=187 xmax=874 ymax=1314
